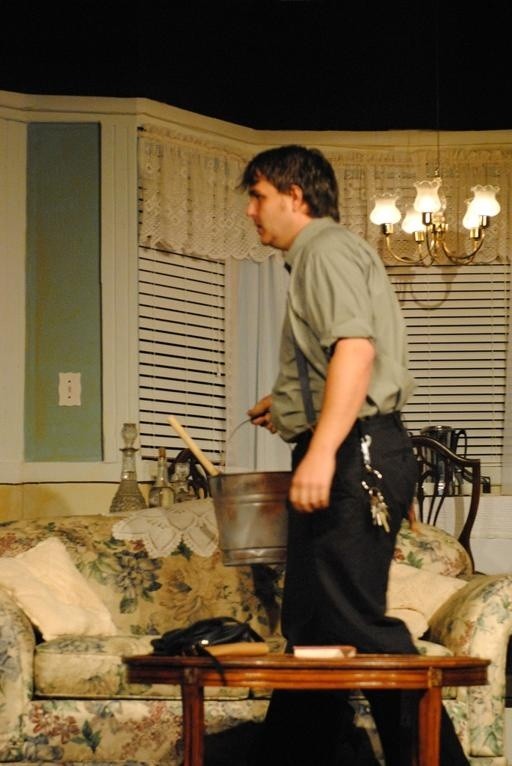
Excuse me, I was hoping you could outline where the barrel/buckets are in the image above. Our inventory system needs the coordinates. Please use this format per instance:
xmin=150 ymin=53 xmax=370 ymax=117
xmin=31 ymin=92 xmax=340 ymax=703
xmin=206 ymin=413 xmax=293 ymax=569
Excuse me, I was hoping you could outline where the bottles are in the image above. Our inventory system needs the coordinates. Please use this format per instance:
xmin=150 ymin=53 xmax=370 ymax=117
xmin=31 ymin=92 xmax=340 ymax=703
xmin=149 ymin=447 xmax=175 ymax=509
xmin=174 ymin=461 xmax=191 ymax=504
xmin=109 ymin=423 xmax=147 ymax=513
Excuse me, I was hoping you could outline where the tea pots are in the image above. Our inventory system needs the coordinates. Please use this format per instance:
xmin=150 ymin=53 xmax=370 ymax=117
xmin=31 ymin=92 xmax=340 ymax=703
xmin=409 ymin=425 xmax=467 ymax=483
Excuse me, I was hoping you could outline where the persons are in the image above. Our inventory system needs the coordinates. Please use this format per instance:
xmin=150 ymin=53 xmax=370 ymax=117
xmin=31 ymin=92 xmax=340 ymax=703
xmin=231 ymin=142 xmax=470 ymax=766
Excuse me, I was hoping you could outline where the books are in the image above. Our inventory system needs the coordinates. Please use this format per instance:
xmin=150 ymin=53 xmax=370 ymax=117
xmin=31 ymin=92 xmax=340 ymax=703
xmin=294 ymin=645 xmax=356 ymax=660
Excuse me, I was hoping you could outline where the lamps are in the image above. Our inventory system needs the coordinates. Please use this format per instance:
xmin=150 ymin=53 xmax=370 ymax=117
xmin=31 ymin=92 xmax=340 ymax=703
xmin=368 ymin=0 xmax=500 ymax=268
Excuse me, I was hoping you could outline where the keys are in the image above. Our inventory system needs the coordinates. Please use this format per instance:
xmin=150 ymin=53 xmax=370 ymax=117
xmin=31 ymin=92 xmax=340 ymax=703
xmin=368 ymin=494 xmax=391 ymax=532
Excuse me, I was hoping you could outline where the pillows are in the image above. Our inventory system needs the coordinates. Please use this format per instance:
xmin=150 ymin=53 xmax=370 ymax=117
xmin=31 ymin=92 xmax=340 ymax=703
xmin=0 ymin=537 xmax=121 ymax=643
xmin=383 ymin=560 xmax=468 ymax=643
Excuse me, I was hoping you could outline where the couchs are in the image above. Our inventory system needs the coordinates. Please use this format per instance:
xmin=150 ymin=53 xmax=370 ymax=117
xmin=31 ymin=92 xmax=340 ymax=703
xmin=0 ymin=498 xmax=508 ymax=766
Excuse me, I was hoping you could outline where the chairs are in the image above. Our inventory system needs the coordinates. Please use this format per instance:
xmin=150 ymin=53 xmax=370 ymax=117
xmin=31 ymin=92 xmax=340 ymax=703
xmin=410 ymin=427 xmax=482 ymax=576
